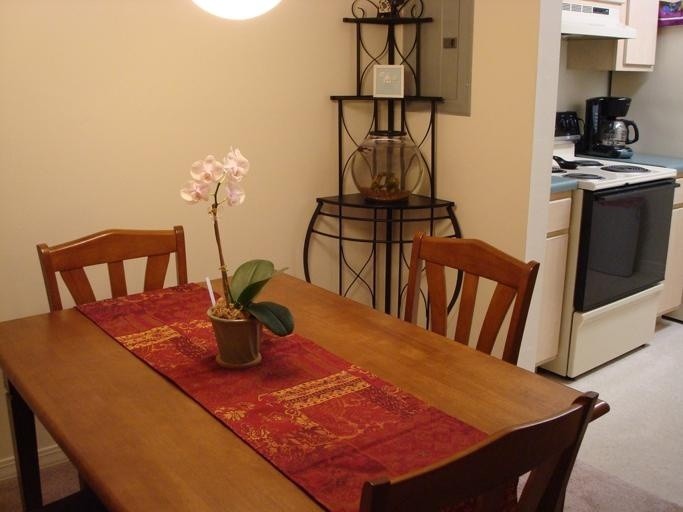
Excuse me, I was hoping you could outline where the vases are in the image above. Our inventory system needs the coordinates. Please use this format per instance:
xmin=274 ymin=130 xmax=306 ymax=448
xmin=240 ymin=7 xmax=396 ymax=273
xmin=203 ymin=302 xmax=262 ymax=370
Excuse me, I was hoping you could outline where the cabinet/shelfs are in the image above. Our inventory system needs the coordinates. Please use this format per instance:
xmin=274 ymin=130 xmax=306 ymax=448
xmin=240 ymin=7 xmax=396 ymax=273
xmin=536 ymin=192 xmax=573 ymax=369
xmin=656 ymin=174 xmax=682 ymax=314
xmin=301 ymin=1 xmax=464 ymax=328
xmin=566 ymin=0 xmax=660 ymax=73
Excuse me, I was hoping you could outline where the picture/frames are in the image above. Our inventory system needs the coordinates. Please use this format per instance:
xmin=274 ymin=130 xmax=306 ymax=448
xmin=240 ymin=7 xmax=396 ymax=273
xmin=372 ymin=64 xmax=405 ymax=100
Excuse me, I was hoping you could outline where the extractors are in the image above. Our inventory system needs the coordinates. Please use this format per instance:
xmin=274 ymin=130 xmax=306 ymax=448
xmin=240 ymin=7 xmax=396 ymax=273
xmin=562 ymin=3 xmax=636 ymax=45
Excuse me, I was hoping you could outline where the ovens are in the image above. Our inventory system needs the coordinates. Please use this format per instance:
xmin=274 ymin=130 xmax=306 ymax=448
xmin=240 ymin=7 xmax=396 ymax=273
xmin=536 ymin=181 xmax=681 ymax=378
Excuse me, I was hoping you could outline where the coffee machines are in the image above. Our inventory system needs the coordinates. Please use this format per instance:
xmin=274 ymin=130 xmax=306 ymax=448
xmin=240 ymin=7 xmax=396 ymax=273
xmin=577 ymin=97 xmax=640 ymax=158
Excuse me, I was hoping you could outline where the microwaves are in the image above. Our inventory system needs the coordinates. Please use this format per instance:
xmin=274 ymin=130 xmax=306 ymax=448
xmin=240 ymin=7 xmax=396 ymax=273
xmin=553 ymin=158 xmax=678 ymax=191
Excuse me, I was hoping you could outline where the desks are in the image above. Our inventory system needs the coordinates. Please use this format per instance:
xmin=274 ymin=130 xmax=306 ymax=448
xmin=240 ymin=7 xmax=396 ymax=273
xmin=0 ymin=268 xmax=607 ymax=511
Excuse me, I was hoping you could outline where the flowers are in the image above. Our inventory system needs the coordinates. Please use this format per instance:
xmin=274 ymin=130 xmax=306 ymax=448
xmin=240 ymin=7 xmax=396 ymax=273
xmin=180 ymin=147 xmax=295 ymax=337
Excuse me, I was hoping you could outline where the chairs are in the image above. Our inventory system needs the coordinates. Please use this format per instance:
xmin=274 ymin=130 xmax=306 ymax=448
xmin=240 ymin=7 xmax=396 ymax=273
xmin=352 ymin=389 xmax=600 ymax=511
xmin=399 ymin=230 xmax=545 ymax=380
xmin=36 ymin=223 xmax=196 ymax=492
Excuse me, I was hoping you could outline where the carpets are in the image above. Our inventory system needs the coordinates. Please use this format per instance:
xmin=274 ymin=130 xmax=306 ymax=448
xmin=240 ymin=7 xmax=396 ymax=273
xmin=516 ymin=454 xmax=683 ymax=511
